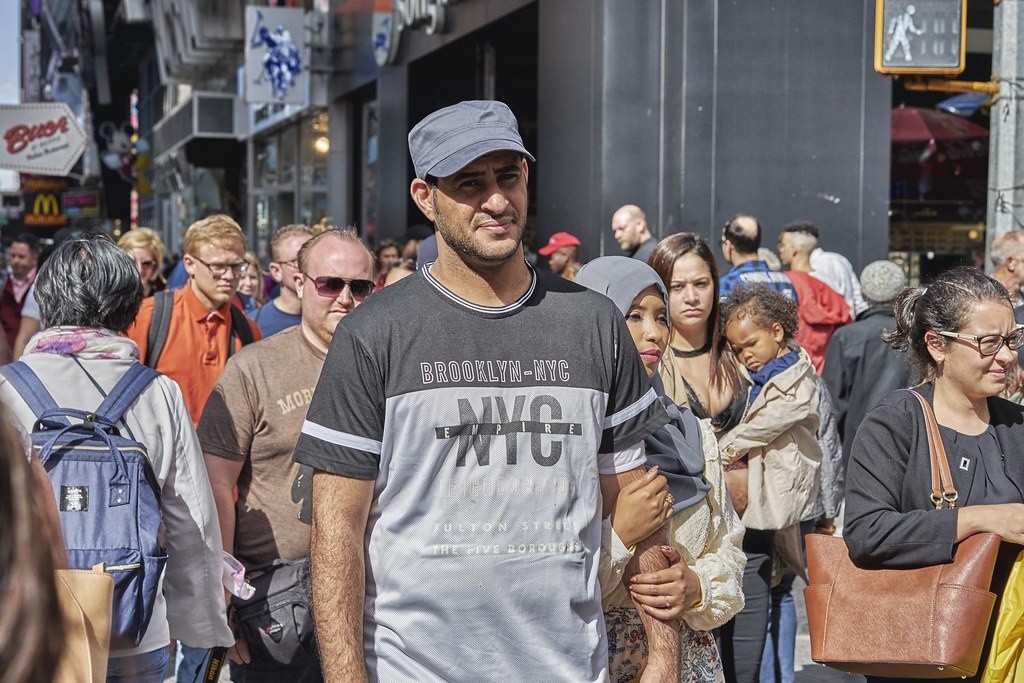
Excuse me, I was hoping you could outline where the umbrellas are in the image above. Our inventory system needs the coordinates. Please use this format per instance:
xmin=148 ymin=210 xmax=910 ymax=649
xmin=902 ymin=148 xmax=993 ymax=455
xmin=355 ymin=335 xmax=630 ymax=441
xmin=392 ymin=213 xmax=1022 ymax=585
xmin=891 ymin=92 xmax=991 ymax=141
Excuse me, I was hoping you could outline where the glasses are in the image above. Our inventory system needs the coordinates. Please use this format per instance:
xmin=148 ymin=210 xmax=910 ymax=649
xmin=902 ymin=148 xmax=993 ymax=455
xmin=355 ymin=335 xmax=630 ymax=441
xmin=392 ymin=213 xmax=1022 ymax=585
xmin=302 ymin=271 xmax=375 ymax=302
xmin=191 ymin=253 xmax=250 ymax=275
xmin=936 ymin=324 xmax=1023 ymax=356
xmin=138 ymin=261 xmax=156 ymax=269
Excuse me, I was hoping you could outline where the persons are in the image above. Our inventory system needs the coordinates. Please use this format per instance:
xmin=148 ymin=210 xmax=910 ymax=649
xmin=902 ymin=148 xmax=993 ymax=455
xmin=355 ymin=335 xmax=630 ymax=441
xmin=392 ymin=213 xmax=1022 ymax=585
xmin=954 ymin=141 xmax=981 ymax=176
xmin=646 ymin=232 xmax=836 ymax=683
xmin=0 ymin=234 xmax=236 ymax=683
xmin=524 ymin=204 xmax=1024 ymax=683
xmin=574 ymin=254 xmax=746 ymax=683
xmin=0 ymin=217 xmax=441 ymax=683
xmin=918 ymin=139 xmax=952 ymax=202
xmin=293 ymin=99 xmax=682 ymax=683
xmin=842 ymin=267 xmax=1024 ymax=683
xmin=713 ymin=280 xmax=845 ymax=530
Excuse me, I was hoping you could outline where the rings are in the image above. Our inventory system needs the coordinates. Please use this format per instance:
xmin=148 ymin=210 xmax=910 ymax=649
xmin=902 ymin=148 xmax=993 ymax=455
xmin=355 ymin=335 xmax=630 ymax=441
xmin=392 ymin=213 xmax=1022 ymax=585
xmin=664 ymin=596 xmax=671 ymax=609
xmin=665 ymin=493 xmax=674 ymax=505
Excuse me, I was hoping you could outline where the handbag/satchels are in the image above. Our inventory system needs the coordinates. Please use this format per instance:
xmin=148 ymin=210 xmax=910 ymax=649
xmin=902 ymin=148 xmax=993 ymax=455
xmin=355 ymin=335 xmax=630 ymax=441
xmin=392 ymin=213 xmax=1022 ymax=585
xmin=803 ymin=389 xmax=1000 ymax=679
xmin=229 ymin=557 xmax=320 ymax=683
xmin=52 ymin=562 xmax=142 ymax=683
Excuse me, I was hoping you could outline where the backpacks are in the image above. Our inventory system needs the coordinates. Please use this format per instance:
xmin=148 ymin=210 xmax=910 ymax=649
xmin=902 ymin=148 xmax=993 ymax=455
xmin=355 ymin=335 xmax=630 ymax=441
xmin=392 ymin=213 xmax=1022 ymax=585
xmin=0 ymin=356 xmax=170 ymax=645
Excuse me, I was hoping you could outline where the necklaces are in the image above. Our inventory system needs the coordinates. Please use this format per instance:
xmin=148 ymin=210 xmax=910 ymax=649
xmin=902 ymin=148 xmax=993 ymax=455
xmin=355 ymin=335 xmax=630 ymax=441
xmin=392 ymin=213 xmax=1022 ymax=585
xmin=669 ymin=333 xmax=715 ymax=359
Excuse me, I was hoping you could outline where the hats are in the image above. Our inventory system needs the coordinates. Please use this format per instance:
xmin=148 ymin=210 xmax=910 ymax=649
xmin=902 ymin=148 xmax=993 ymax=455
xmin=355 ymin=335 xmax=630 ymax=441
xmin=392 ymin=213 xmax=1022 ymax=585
xmin=860 ymin=259 xmax=905 ymax=302
xmin=395 ymin=227 xmax=434 ymax=244
xmin=408 ymin=100 xmax=535 ymax=182
xmin=415 ymin=234 xmax=441 ymax=271
xmin=538 ymin=233 xmax=582 ymax=256
